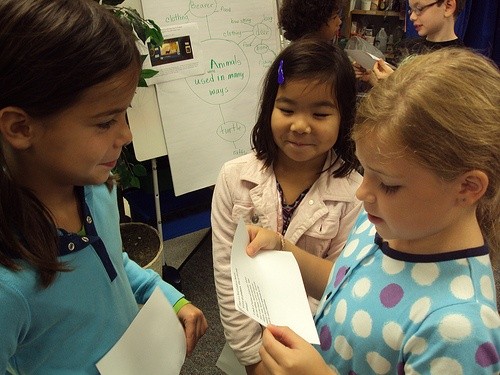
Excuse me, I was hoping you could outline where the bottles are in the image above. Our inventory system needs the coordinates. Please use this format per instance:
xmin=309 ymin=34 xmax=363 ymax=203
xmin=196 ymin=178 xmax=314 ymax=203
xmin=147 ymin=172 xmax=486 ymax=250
xmin=375 ymin=28 xmax=387 ymax=54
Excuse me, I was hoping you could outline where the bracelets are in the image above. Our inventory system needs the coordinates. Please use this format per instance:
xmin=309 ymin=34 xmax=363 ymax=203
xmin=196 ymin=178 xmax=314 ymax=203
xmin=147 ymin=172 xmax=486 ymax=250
xmin=277 ymin=231 xmax=285 ymax=251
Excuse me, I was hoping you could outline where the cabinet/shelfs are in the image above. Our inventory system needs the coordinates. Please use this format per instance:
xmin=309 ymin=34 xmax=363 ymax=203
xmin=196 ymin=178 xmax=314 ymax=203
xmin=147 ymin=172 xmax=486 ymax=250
xmin=344 ymin=0 xmax=409 ymax=63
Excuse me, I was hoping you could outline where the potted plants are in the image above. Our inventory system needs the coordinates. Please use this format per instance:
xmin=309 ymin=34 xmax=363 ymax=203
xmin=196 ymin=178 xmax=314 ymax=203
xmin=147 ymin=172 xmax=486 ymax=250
xmin=98 ymin=0 xmax=163 ymax=278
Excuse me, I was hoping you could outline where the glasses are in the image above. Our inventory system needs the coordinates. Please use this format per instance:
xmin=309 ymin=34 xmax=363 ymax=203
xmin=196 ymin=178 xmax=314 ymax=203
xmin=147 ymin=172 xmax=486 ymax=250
xmin=408 ymin=1 xmax=444 ymax=15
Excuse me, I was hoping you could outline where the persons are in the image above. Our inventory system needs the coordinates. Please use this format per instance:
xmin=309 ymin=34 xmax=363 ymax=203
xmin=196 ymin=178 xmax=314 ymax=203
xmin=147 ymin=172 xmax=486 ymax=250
xmin=0 ymin=0 xmax=210 ymax=375
xmin=211 ymin=0 xmax=500 ymax=375
